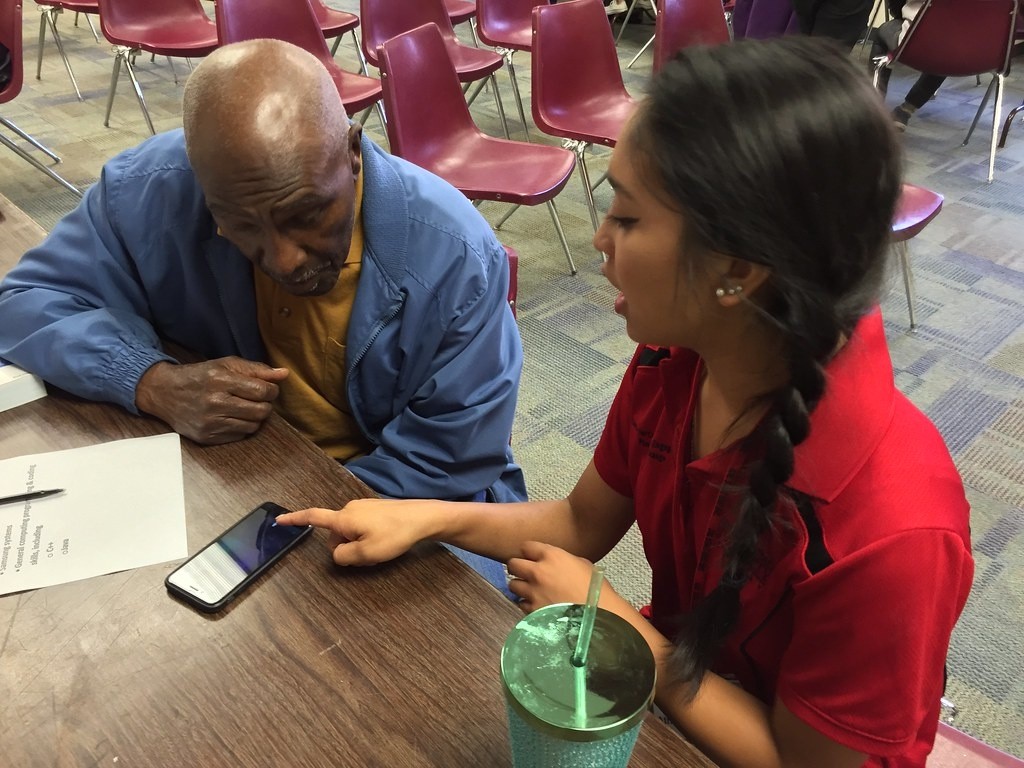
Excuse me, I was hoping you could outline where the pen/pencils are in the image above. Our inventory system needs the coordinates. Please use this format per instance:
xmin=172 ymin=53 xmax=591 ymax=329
xmin=0 ymin=488 xmax=63 ymax=505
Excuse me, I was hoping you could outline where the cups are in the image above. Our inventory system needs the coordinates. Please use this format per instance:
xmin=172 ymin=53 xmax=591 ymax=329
xmin=501 ymin=602 xmax=657 ymax=768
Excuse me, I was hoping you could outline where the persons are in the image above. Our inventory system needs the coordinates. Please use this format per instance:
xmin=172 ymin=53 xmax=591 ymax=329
xmin=870 ymin=0 xmax=952 ymax=132
xmin=0 ymin=39 xmax=529 ymax=599
xmin=273 ymin=35 xmax=1024 ymax=768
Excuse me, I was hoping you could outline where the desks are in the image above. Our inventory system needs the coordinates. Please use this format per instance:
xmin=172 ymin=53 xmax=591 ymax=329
xmin=1 ymin=355 xmax=724 ymax=768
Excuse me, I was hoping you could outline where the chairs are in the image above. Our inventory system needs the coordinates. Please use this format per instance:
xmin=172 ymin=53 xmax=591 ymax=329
xmin=0 ymin=0 xmax=1024 ymax=332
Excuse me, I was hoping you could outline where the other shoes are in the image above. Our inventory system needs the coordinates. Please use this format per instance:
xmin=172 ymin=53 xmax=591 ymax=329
xmin=888 ymin=106 xmax=911 ymax=133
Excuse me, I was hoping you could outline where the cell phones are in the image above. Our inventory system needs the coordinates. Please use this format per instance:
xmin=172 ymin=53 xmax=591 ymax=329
xmin=164 ymin=501 xmax=316 ymax=615
